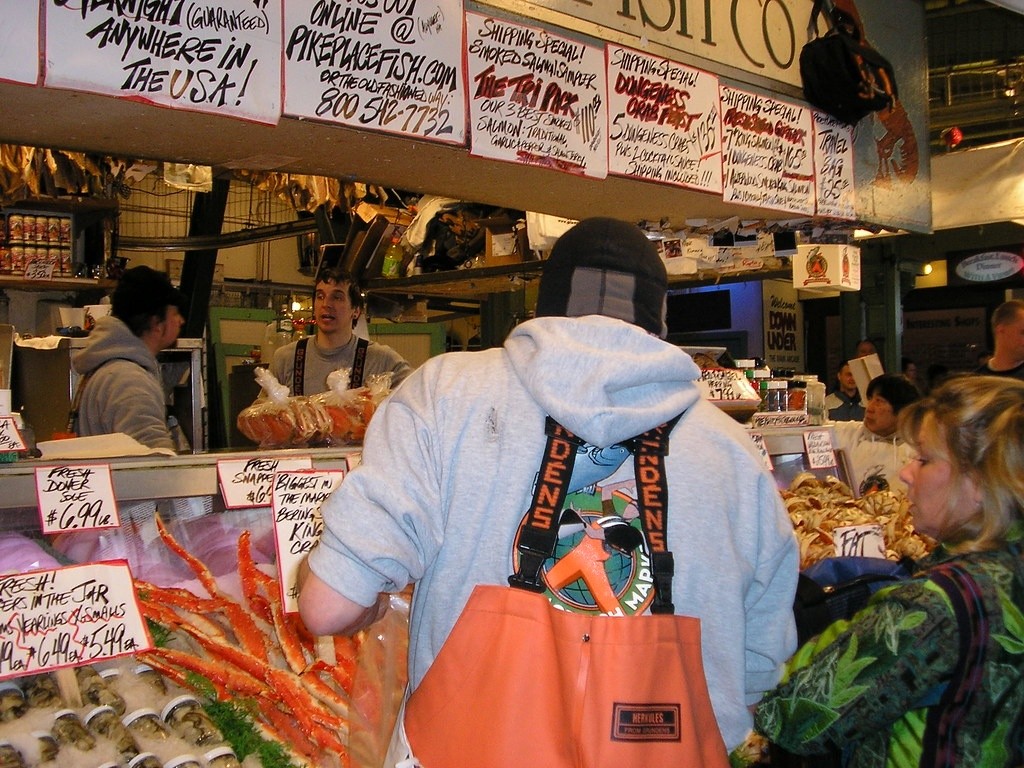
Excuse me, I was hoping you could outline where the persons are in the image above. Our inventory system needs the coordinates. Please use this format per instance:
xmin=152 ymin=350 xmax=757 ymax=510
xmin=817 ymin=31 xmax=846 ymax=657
xmin=729 ymin=374 xmax=1024 ymax=768
xmin=253 ymin=269 xmax=417 ymax=408
xmin=72 ymin=266 xmax=186 ymax=453
xmin=295 ymin=214 xmax=802 ymax=768
xmin=824 ymin=298 xmax=1024 ymax=504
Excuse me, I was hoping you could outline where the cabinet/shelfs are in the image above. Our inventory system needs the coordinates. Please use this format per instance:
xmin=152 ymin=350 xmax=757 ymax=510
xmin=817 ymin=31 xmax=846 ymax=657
xmin=0 ymin=192 xmax=118 ymax=290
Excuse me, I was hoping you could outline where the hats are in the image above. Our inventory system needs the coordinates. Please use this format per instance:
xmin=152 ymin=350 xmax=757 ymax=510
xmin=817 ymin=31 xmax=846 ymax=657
xmin=536 ymin=216 xmax=667 ymax=335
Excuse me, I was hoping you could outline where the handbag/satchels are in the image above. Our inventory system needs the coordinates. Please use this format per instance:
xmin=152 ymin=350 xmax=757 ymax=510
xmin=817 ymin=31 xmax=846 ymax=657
xmin=800 ymin=0 xmax=897 ymax=125
xmin=794 ymin=556 xmax=915 ymax=653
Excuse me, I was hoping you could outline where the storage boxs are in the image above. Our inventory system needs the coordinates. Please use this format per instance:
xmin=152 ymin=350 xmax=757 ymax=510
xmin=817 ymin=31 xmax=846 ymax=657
xmin=474 ymin=217 xmax=528 ymax=266
xmin=338 ymin=202 xmax=413 ymax=275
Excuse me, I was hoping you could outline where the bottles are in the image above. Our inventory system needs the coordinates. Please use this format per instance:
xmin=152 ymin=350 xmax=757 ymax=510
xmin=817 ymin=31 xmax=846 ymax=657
xmin=382 ymin=238 xmax=403 ymax=278
xmin=744 ymin=369 xmax=827 ymax=426
xmin=277 ymin=304 xmax=293 ymax=348
xmin=415 ymin=254 xmax=424 ymax=275
